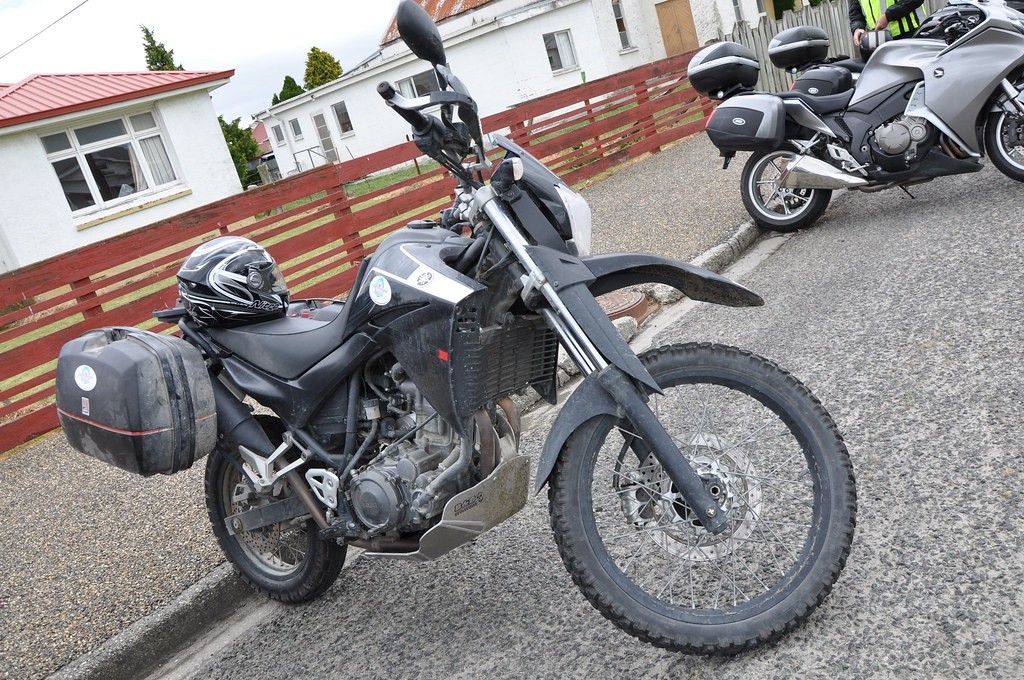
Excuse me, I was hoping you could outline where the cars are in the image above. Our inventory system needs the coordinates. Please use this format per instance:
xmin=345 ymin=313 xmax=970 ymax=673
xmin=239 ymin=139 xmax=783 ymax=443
xmin=240 ymin=153 xmax=280 ymax=186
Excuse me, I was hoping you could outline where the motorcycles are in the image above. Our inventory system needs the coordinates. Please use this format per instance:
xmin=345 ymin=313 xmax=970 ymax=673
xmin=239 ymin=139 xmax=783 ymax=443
xmin=691 ymin=0 xmax=1024 ymax=232
xmin=53 ymin=0 xmax=858 ymax=652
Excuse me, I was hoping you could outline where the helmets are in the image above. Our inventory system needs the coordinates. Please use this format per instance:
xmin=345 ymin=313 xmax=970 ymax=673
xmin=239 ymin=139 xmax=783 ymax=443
xmin=859 ymin=29 xmax=893 ymax=64
xmin=175 ymin=236 xmax=289 ymax=331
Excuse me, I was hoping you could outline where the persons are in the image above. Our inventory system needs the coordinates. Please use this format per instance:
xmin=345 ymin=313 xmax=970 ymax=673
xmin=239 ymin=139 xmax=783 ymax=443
xmin=847 ymin=0 xmax=928 ymax=63
